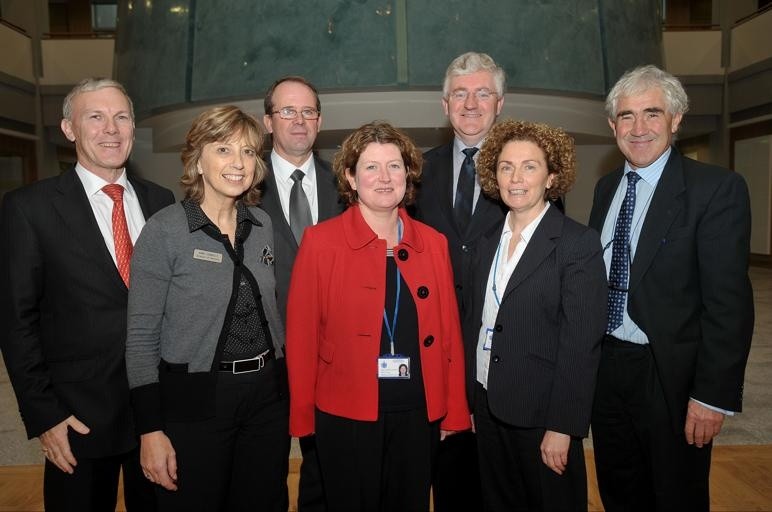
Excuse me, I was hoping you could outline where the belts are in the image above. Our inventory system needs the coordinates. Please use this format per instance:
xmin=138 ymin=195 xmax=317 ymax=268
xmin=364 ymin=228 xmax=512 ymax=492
xmin=216 ymin=348 xmax=271 ymax=375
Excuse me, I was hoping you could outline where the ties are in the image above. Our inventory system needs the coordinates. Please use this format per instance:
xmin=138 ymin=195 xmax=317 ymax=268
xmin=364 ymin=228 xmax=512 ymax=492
xmin=102 ymin=183 xmax=133 ymax=290
xmin=606 ymin=171 xmax=641 ymax=335
xmin=454 ymin=147 xmax=480 ymax=233
xmin=289 ymin=170 xmax=313 ymax=247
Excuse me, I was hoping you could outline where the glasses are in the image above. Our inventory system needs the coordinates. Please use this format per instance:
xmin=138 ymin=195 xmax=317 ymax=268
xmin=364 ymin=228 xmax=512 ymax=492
xmin=447 ymin=87 xmax=499 ymax=103
xmin=267 ymin=105 xmax=320 ymax=121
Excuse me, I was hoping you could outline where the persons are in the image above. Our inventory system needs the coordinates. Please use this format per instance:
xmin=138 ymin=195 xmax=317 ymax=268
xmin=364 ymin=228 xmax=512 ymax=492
xmin=125 ymin=104 xmax=288 ymax=511
xmin=587 ymin=66 xmax=755 ymax=511
xmin=1 ymin=76 xmax=176 ymax=512
xmin=258 ymin=77 xmax=345 ymax=512
xmin=286 ymin=118 xmax=472 ymax=512
xmin=410 ymin=52 xmax=564 ymax=511
xmin=399 ymin=363 xmax=409 ymax=379
xmin=461 ymin=115 xmax=608 ymax=511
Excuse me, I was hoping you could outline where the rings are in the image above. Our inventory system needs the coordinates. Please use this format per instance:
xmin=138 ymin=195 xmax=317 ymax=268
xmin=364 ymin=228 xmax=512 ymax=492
xmin=145 ymin=474 xmax=153 ymax=479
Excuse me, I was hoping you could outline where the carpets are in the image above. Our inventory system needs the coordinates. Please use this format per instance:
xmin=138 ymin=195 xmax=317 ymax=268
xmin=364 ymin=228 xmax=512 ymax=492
xmin=1 ymin=265 xmax=772 ymax=467
